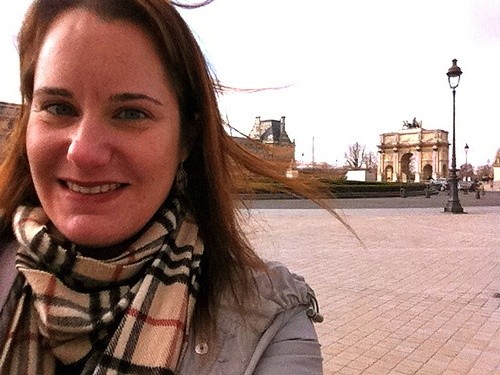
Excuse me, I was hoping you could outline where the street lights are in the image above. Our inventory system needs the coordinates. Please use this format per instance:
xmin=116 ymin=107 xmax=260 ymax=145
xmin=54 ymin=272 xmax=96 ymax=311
xmin=445 ymin=58 xmax=465 ymax=214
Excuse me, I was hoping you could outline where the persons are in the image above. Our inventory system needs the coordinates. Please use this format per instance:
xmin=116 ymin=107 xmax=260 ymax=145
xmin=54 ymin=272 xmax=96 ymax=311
xmin=1 ymin=0 xmax=333 ymax=375
